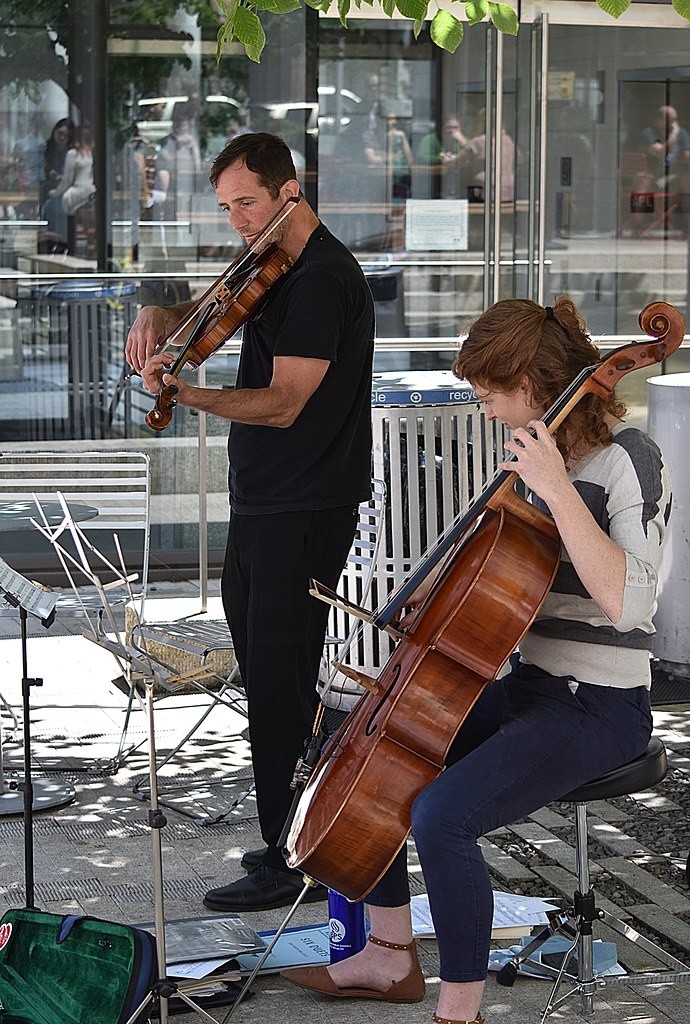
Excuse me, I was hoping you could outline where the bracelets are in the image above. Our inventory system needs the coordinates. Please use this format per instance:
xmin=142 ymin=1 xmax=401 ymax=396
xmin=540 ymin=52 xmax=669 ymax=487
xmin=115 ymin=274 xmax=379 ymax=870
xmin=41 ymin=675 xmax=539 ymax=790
xmin=162 ymin=305 xmax=185 ymax=322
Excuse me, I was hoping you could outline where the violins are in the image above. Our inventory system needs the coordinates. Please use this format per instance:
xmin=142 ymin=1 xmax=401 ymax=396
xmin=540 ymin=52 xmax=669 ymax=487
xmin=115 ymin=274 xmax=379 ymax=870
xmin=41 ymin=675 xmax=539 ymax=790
xmin=144 ymin=244 xmax=293 ymax=431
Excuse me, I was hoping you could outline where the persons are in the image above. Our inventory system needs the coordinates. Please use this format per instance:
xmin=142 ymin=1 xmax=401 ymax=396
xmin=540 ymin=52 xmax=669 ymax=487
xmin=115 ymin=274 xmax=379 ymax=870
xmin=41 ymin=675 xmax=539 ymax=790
xmin=122 ymin=133 xmax=377 ymax=914
xmin=279 ymin=293 xmax=675 ymax=1024
xmin=0 ymin=91 xmax=337 ymax=260
xmin=335 ymin=70 xmax=690 ymax=242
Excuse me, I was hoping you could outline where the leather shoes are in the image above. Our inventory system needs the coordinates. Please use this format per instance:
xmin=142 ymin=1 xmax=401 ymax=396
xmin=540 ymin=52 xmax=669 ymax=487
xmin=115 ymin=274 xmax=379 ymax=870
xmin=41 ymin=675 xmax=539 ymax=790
xmin=431 ymin=1011 xmax=485 ymax=1024
xmin=240 ymin=846 xmax=305 ymax=875
xmin=203 ymin=865 xmax=328 ymax=912
xmin=279 ymin=933 xmax=426 ymax=1003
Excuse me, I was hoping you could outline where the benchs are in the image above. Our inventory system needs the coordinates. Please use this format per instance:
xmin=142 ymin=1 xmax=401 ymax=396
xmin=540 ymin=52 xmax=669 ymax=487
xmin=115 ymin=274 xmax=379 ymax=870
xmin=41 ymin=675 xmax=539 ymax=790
xmin=614 ymin=189 xmax=688 ymax=238
xmin=88 ymin=199 xmax=540 ymax=253
xmin=0 ymin=192 xmax=39 ymax=220
xmin=0 ymin=452 xmax=150 ymax=627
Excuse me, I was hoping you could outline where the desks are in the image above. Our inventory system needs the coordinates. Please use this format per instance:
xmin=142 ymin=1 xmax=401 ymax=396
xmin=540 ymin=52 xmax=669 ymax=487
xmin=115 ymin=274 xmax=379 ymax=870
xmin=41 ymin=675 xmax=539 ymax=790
xmin=88 ymin=162 xmax=448 ymax=199
xmin=0 ymin=502 xmax=100 ymax=532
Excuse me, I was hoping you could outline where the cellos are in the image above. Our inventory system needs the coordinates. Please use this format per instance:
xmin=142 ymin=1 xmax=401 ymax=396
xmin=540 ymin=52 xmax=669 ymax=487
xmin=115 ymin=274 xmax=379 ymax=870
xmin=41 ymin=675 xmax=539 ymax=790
xmin=222 ymin=306 xmax=681 ymax=1024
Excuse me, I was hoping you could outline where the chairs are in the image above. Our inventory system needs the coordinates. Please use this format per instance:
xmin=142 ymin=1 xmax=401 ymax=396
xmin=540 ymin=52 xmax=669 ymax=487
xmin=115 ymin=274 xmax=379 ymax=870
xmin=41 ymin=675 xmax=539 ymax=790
xmin=131 ymin=480 xmax=387 ymax=826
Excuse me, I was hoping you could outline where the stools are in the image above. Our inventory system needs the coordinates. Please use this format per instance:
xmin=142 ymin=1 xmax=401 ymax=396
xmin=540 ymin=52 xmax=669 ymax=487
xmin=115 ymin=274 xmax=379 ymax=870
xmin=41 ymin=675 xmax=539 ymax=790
xmin=498 ymin=736 xmax=690 ymax=1024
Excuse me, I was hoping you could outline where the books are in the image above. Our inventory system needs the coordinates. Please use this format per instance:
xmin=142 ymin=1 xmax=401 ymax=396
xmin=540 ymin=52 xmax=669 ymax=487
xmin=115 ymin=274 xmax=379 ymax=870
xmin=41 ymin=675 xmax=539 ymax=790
xmin=234 ymin=916 xmax=372 ymax=976
xmin=127 ymin=913 xmax=273 ymax=999
